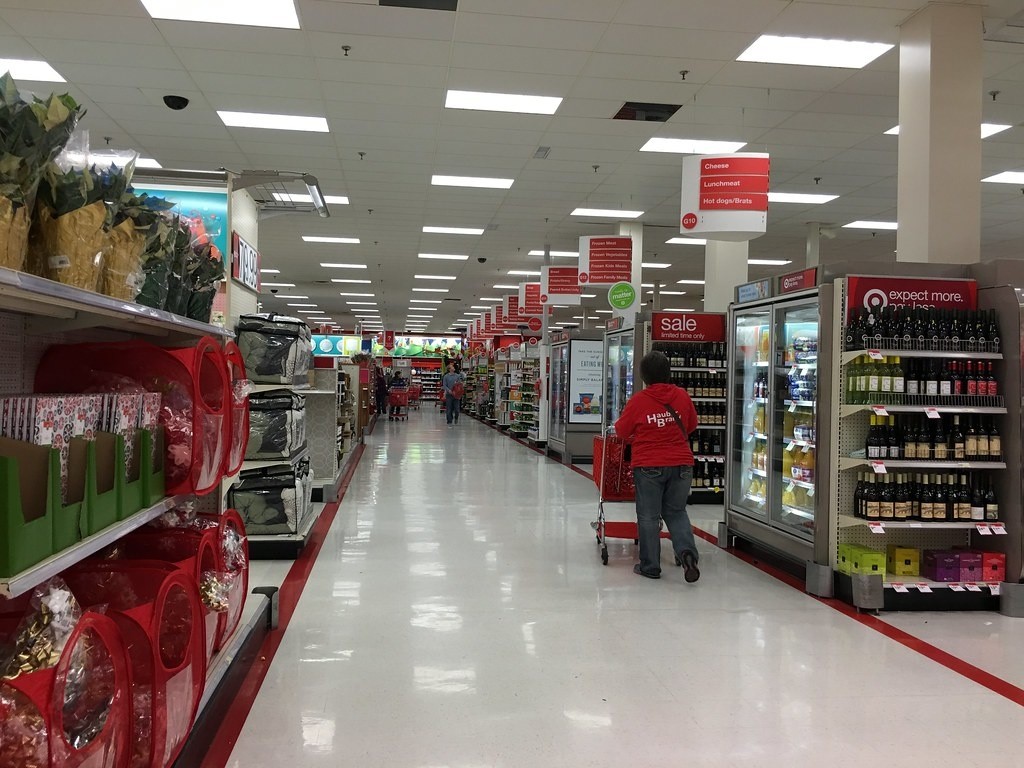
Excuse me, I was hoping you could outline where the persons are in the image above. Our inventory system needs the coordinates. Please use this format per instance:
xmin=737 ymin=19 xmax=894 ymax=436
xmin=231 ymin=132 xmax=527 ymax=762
xmin=376 ymin=372 xmax=387 ymax=415
xmin=388 ymin=371 xmax=404 ymax=421
xmin=443 ymin=364 xmax=461 ymax=429
xmin=615 ymin=351 xmax=700 ymax=584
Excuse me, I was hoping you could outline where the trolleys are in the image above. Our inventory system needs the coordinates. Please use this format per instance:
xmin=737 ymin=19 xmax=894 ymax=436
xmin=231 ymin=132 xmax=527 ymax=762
xmin=590 ymin=424 xmax=682 ymax=565
xmin=388 ymin=387 xmax=409 ymax=422
xmin=406 ymin=384 xmax=420 ymax=411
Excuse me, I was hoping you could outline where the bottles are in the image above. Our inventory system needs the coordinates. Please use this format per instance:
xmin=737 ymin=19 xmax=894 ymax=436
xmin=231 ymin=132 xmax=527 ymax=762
xmin=654 ymin=344 xmax=725 ymax=488
xmin=750 ymin=327 xmax=814 ymax=510
xmin=849 ymin=307 xmax=1002 ymax=520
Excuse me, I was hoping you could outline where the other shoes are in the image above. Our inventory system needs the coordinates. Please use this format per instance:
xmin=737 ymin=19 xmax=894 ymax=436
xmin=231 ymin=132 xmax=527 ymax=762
xmin=633 ymin=563 xmax=661 ymax=579
xmin=680 ymin=550 xmax=700 ymax=583
xmin=454 ymin=416 xmax=458 ymax=424
xmin=448 ymin=422 xmax=453 ymax=429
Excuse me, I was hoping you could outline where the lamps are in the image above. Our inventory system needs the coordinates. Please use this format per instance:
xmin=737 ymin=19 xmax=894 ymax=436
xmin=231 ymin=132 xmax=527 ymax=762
xmin=304 ymin=175 xmax=330 ymax=219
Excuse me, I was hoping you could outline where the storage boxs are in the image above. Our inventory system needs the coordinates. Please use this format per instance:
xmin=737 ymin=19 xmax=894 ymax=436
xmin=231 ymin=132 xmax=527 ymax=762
xmin=839 ymin=544 xmax=1007 ymax=583
xmin=0 ymin=427 xmax=166 ymax=579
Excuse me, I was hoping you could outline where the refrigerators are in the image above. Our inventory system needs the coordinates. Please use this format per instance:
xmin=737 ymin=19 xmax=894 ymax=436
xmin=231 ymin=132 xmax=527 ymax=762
xmin=716 ymin=284 xmax=831 ymax=577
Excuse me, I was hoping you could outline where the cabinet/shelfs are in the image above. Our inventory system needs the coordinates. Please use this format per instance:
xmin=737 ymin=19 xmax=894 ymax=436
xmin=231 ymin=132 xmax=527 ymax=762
xmin=638 ymin=320 xmax=727 ymax=506
xmin=219 ymin=383 xmax=319 ymax=560
xmin=0 ymin=266 xmax=280 ymax=768
xmin=411 ymin=341 xmax=539 ymax=441
xmin=830 ymin=280 xmax=1010 ymax=612
xmin=305 ymin=356 xmax=383 ymax=502
